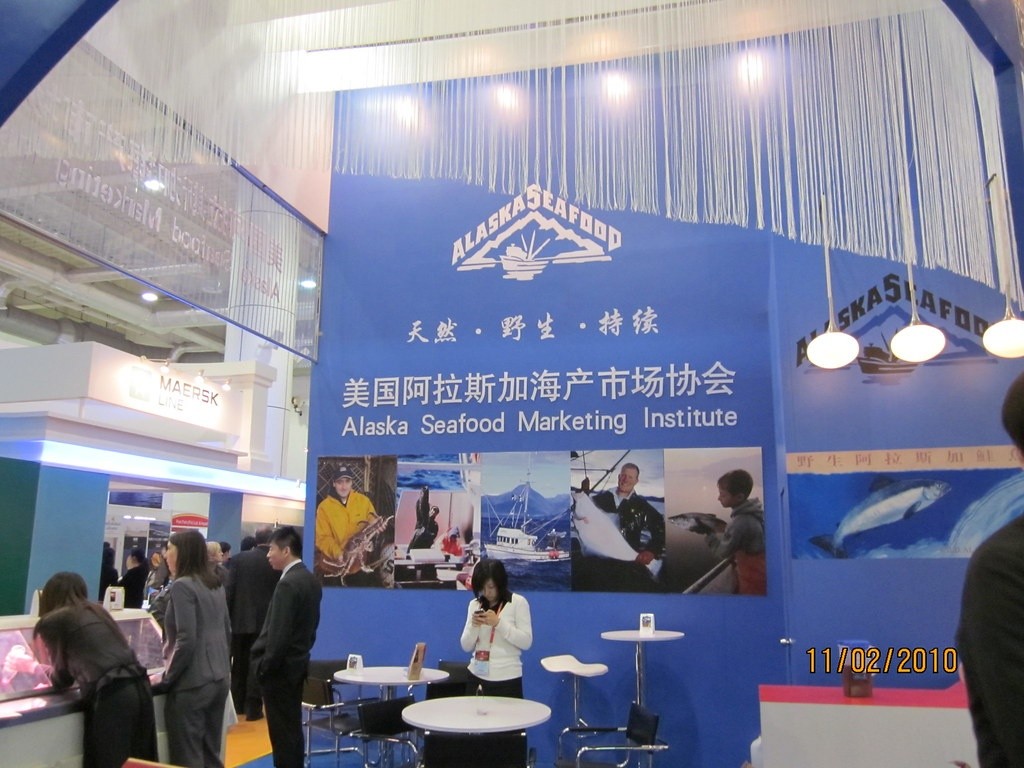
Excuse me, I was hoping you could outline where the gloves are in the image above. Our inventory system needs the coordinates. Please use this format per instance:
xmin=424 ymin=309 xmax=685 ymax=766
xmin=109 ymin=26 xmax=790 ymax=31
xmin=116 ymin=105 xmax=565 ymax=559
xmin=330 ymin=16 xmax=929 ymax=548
xmin=688 ymin=516 xmax=714 ymax=535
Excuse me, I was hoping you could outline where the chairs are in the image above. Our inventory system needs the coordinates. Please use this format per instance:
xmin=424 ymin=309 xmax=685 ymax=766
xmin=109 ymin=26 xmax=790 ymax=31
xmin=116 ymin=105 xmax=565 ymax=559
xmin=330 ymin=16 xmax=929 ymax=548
xmin=301 ymin=660 xmax=471 ymax=768
xmin=555 ymin=700 xmax=670 ymax=768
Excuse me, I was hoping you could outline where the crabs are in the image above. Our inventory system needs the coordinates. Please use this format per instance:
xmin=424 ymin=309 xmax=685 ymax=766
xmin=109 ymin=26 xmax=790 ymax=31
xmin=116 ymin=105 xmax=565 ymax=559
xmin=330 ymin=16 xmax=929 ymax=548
xmin=321 ymin=512 xmax=393 ymax=590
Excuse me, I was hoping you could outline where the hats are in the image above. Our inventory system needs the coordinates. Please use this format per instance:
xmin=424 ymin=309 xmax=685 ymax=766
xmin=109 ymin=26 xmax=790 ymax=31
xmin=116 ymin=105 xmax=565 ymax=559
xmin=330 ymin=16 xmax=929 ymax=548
xmin=332 ymin=465 xmax=353 ymax=483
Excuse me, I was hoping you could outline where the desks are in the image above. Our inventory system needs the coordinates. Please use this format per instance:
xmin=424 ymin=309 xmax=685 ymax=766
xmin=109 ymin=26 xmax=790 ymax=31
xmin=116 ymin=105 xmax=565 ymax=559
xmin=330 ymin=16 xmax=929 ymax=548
xmin=334 ymin=666 xmax=450 ymax=768
xmin=600 ymin=630 xmax=686 ymax=768
xmin=403 ymin=695 xmax=552 ymax=734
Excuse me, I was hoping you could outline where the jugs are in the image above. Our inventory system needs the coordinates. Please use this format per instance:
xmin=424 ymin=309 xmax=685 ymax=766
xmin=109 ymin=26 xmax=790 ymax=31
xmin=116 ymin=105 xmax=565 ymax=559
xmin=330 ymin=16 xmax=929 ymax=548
xmin=836 ymin=639 xmax=877 ymax=697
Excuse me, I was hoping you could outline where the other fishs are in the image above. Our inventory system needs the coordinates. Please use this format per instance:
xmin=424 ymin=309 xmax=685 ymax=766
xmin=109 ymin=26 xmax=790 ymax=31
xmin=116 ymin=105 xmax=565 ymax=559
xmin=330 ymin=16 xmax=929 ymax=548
xmin=570 ymin=490 xmax=666 ymax=582
xmin=667 ymin=513 xmax=728 ymax=536
xmin=807 ymin=471 xmax=954 ymax=564
xmin=407 ymin=486 xmax=431 ymax=558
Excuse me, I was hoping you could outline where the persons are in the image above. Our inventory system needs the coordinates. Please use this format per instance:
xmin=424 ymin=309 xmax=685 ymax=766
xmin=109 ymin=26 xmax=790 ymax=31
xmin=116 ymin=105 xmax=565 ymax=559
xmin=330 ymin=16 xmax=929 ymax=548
xmin=955 ymin=373 xmax=1024 ymax=768
xmin=459 ymin=559 xmax=533 ymax=699
xmin=8 ymin=570 xmax=159 ymax=768
xmin=690 ymin=469 xmax=764 ymax=595
xmin=441 ymin=527 xmax=464 ymax=557
xmin=98 ymin=525 xmax=323 ymax=767
xmin=407 ymin=486 xmax=440 ymax=556
xmin=315 ymin=466 xmax=377 ymax=587
xmin=574 ymin=462 xmax=666 ymax=591
xmin=461 ymin=529 xmax=482 ymax=563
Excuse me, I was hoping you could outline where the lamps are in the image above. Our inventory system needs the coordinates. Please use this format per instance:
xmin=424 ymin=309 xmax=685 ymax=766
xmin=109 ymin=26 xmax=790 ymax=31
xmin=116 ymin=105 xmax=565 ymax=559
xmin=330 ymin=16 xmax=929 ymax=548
xmin=806 ymin=175 xmax=1024 ymax=370
xmin=161 ymin=360 xmax=170 ymax=373
xmin=194 ymin=369 xmax=205 ymax=384
xmin=222 ymin=377 xmax=232 ymax=391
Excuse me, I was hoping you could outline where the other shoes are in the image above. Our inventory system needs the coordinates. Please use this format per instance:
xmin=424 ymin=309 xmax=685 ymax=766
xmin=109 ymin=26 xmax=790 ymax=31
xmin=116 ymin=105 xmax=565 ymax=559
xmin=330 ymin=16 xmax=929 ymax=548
xmin=246 ymin=713 xmax=263 ymax=721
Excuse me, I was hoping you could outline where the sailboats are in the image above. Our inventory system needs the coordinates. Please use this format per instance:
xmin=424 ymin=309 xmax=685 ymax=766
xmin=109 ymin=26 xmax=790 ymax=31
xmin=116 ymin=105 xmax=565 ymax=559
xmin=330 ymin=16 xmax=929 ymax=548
xmin=486 ymin=469 xmax=570 ymax=562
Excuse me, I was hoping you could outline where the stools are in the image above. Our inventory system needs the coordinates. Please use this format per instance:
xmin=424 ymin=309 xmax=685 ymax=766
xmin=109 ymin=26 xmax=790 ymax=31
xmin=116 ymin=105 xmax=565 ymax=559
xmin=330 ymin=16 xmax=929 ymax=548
xmin=540 ymin=655 xmax=609 ymax=750
